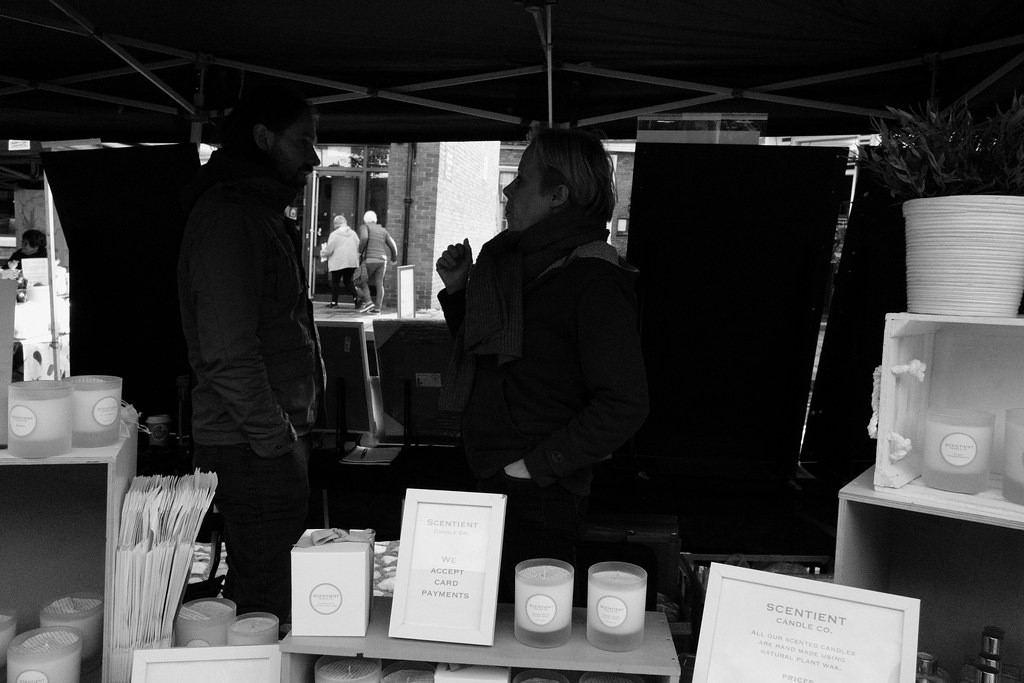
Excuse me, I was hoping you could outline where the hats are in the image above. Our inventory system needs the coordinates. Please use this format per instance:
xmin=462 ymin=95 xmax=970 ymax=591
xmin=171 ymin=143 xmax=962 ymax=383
xmin=364 ymin=211 xmax=378 ymax=224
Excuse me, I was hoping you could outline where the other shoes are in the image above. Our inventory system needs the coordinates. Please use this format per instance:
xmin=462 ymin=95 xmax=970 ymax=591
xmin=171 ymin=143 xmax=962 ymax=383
xmin=367 ymin=308 xmax=381 ymax=315
xmin=325 ymin=302 xmax=339 ymax=309
xmin=359 ymin=301 xmax=375 ymax=314
xmin=352 ymin=297 xmax=363 ymax=309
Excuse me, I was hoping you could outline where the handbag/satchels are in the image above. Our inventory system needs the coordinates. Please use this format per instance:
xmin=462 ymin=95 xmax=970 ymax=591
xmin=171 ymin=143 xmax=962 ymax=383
xmin=351 ymin=261 xmax=369 ymax=289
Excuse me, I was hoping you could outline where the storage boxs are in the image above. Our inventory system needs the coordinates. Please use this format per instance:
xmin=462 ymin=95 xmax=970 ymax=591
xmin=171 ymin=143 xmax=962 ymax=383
xmin=291 ymin=529 xmax=374 ymax=636
xmin=872 ymin=310 xmax=1024 ymax=515
xmin=832 ymin=464 xmax=1024 ymax=683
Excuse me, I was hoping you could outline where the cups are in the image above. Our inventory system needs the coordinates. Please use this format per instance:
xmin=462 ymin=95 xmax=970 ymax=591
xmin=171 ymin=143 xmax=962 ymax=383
xmin=7 ymin=381 xmax=72 ymax=458
xmin=0 ymin=558 xmax=648 ymax=683
xmin=145 ymin=414 xmax=171 ymax=446
xmin=1003 ymin=408 xmax=1024 ymax=505
xmin=63 ymin=375 xmax=122 ymax=447
xmin=923 ymin=407 xmax=996 ymax=494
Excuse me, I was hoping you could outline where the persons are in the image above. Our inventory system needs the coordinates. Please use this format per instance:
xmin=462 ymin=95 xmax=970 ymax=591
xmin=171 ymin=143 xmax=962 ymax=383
xmin=2 ymin=229 xmax=47 ymax=269
xmin=320 ymin=210 xmax=398 ymax=315
xmin=178 ymin=97 xmax=327 ymax=635
xmin=436 ymin=124 xmax=650 ymax=602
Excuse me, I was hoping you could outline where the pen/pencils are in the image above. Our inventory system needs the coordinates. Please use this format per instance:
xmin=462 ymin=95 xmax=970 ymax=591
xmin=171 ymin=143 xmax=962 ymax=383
xmin=360 ymin=448 xmax=369 ymax=459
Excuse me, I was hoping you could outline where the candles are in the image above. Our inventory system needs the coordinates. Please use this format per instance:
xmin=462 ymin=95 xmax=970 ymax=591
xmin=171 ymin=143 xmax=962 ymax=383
xmin=314 ymin=655 xmax=436 ymax=683
xmin=586 ymin=561 xmax=648 ymax=652
xmin=514 ymin=557 xmax=574 ymax=648
xmin=0 ymin=591 xmax=103 ymax=683
xmin=999 ymin=411 xmax=1024 ymax=503
xmin=924 ymin=399 xmax=994 ymax=493
xmin=175 ymin=598 xmax=279 ymax=646
xmin=10 ymin=369 xmax=122 ymax=458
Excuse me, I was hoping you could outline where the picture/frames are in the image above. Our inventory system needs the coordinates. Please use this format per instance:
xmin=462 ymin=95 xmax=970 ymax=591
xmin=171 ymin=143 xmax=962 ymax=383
xmin=131 ymin=644 xmax=282 ymax=683
xmin=390 ymin=487 xmax=510 ymax=646
xmin=690 ymin=561 xmax=921 ymax=683
